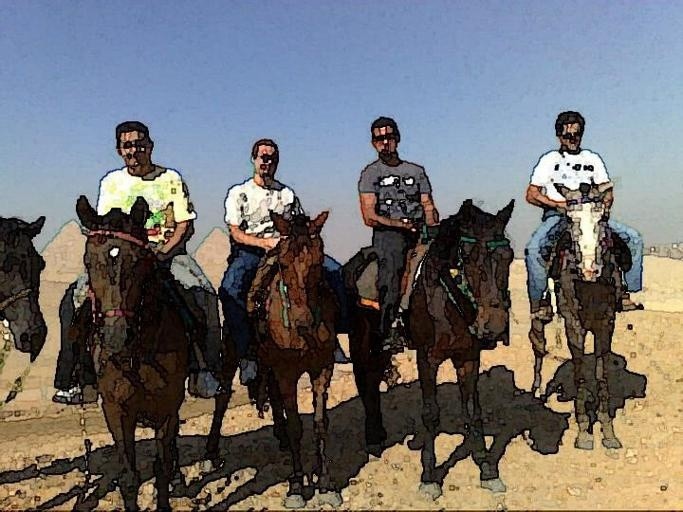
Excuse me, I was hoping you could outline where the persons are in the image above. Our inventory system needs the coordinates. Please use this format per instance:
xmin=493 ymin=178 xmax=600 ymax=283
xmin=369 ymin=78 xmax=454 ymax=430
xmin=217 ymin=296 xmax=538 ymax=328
xmin=52 ymin=121 xmax=221 ymax=404
xmin=357 ymin=115 xmax=440 ymax=353
xmin=217 ymin=138 xmax=353 ymax=386
xmin=524 ymin=110 xmax=645 ymax=322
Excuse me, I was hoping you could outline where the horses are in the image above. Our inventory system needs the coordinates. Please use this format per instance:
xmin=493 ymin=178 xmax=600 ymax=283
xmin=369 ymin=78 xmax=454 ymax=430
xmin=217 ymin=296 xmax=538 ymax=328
xmin=58 ymin=194 xmax=194 ymax=511
xmin=529 ymin=181 xmax=623 ymax=451
xmin=205 ymin=210 xmax=343 ymax=510
xmin=341 ymin=198 xmax=516 ymax=502
xmin=0 ymin=214 xmax=48 ymax=355
